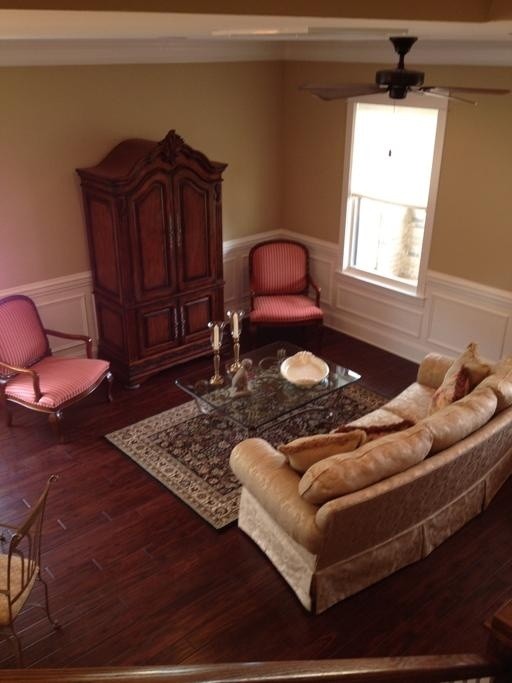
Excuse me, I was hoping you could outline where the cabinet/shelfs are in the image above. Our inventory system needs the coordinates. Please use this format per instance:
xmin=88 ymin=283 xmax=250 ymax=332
xmin=75 ymin=129 xmax=229 ymax=388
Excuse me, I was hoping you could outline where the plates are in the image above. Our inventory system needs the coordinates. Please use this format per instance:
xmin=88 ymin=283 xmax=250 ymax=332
xmin=279 ymin=349 xmax=330 ymax=385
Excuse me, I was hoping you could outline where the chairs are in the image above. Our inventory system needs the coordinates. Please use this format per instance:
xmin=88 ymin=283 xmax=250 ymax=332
xmin=0 ymin=293 xmax=115 ymax=443
xmin=1 ymin=473 xmax=63 ymax=670
xmin=247 ymin=237 xmax=325 ymax=342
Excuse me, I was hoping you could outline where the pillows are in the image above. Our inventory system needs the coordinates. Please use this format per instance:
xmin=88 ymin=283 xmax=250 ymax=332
xmin=431 ymin=342 xmax=496 ymax=415
xmin=278 ymin=419 xmax=415 ymax=473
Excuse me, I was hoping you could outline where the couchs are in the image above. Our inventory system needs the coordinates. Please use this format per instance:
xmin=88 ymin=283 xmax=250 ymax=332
xmin=229 ymin=354 xmax=511 ymax=616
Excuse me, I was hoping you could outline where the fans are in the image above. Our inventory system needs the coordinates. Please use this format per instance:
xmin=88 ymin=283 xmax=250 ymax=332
xmin=303 ymin=34 xmax=511 ymax=107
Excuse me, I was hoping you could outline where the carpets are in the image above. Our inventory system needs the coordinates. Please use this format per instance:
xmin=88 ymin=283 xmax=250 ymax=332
xmin=106 ymin=359 xmax=394 ymax=529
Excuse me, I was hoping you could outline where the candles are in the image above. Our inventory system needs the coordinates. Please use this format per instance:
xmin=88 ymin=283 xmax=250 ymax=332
xmin=214 ymin=324 xmax=219 ymax=346
xmin=233 ymin=311 xmax=239 ymax=337
